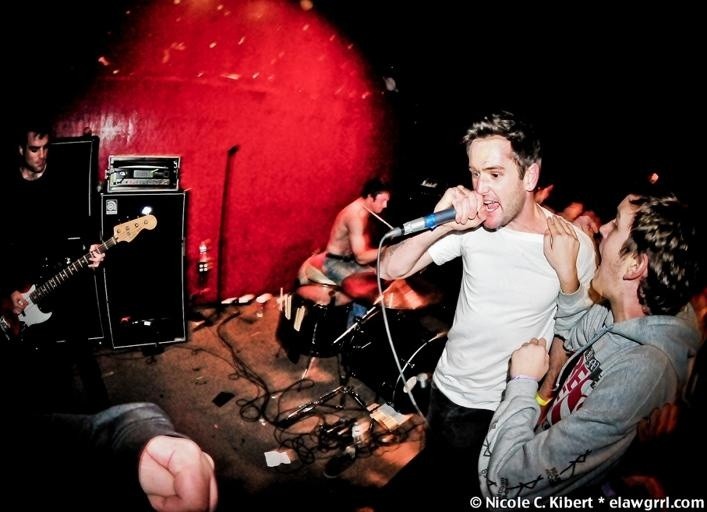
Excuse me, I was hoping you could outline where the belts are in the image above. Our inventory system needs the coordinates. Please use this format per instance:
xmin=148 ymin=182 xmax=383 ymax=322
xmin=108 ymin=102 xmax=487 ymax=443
xmin=326 ymin=253 xmax=354 ymax=262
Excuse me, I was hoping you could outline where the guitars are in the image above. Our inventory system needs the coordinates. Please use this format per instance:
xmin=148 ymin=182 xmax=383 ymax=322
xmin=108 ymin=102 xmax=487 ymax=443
xmin=2 ymin=210 xmax=157 ymax=346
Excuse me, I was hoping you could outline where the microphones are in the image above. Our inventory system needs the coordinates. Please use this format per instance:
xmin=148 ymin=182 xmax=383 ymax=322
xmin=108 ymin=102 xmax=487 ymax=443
xmin=391 ymin=208 xmax=456 ymax=242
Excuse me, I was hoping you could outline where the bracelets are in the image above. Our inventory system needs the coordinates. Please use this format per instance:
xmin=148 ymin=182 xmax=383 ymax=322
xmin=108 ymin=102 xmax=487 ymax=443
xmin=535 ymin=392 xmax=554 ymax=407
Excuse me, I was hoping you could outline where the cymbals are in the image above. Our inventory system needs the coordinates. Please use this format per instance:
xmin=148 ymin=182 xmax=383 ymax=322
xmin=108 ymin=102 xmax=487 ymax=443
xmin=344 ymin=272 xmax=449 ymax=310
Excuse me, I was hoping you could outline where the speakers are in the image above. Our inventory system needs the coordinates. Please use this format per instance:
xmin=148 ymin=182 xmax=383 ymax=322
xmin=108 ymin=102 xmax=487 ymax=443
xmin=100 ymin=189 xmax=189 ymax=350
xmin=48 ymin=134 xmax=110 ymax=350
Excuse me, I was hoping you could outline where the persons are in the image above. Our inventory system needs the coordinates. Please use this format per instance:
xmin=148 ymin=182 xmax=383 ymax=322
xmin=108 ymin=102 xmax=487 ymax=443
xmin=599 ymin=400 xmax=707 ymax=510
xmin=475 ymin=185 xmax=706 ymax=511
xmin=0 ymin=121 xmax=111 ymax=416
xmin=322 ymin=177 xmax=390 ymax=330
xmin=373 ymin=109 xmax=600 ymax=512
xmin=0 ymin=398 xmax=220 ymax=512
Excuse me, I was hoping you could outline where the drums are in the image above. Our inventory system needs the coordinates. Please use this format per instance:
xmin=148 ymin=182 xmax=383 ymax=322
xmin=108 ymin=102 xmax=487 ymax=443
xmin=349 ymin=303 xmax=457 ymax=414
xmin=288 ymin=285 xmax=353 ymax=359
